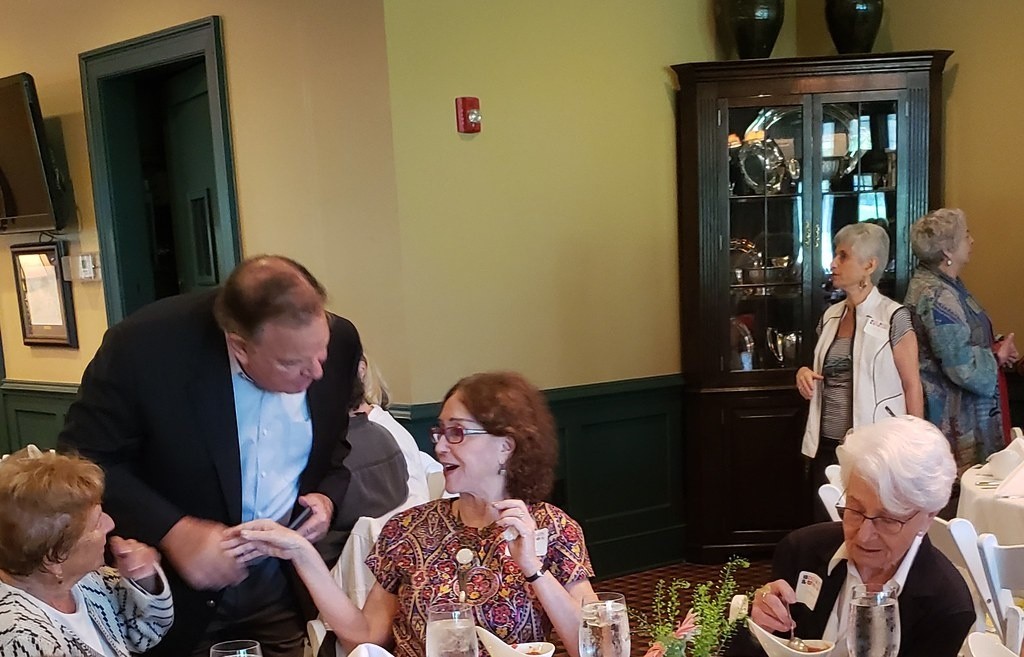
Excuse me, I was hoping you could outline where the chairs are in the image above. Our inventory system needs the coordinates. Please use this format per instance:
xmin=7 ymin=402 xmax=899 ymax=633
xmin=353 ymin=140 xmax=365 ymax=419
xmin=816 ymin=465 xmax=849 ymax=522
xmin=976 ymin=529 xmax=1024 ymax=644
xmin=927 ymin=516 xmax=1015 ymax=639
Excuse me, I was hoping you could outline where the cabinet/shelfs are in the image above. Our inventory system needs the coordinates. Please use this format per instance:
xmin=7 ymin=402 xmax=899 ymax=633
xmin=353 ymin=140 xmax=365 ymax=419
xmin=669 ymin=48 xmax=955 ymax=566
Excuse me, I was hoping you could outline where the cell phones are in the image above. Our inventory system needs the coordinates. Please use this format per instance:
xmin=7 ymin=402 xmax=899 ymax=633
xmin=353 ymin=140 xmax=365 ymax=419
xmin=288 ymin=507 xmax=312 ymax=530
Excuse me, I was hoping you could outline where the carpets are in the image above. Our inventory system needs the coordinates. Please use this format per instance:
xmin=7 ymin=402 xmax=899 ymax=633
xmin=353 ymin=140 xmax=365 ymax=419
xmin=623 ymin=558 xmax=773 ymax=657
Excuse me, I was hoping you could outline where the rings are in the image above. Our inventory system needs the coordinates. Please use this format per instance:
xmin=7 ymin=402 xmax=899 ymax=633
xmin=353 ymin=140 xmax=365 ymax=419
xmin=760 ymin=591 xmax=769 ymax=602
xmin=798 ymin=384 xmax=804 ymax=392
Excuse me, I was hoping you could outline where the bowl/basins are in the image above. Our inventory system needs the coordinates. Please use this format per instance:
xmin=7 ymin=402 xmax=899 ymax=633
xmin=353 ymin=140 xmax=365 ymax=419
xmin=964 ymin=632 xmax=1022 ymax=657
xmin=747 ymin=618 xmax=835 ymax=657
xmin=475 ymin=626 xmax=556 ymax=657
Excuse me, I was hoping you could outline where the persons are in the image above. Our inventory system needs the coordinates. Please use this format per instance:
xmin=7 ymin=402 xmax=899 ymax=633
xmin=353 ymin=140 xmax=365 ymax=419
xmin=0 ymin=447 xmax=173 ymax=657
xmin=56 ymin=255 xmax=364 ymax=657
xmin=903 ymin=208 xmax=1019 ymax=496
xmin=313 ymin=353 xmax=431 ymax=571
xmin=219 ymin=370 xmax=617 ymax=657
xmin=724 ymin=415 xmax=977 ymax=657
xmin=796 ymin=223 xmax=923 ymax=525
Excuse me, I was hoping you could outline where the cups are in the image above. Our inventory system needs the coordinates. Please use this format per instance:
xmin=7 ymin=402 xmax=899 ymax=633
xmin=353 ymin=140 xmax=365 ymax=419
xmin=846 ymin=584 xmax=901 ymax=657
xmin=210 ymin=640 xmax=263 ymax=657
xmin=579 ymin=592 xmax=631 ymax=657
xmin=425 ymin=603 xmax=479 ymax=657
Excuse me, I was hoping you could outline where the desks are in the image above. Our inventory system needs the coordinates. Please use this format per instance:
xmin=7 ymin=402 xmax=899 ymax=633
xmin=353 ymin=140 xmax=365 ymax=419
xmin=957 ymin=458 xmax=1024 ymax=600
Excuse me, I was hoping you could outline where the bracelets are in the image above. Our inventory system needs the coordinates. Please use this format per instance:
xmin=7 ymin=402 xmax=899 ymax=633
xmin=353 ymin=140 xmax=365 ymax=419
xmin=524 ymin=561 xmax=549 ymax=583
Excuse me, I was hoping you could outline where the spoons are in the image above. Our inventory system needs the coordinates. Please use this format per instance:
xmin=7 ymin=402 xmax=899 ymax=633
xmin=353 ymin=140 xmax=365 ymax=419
xmin=780 ymin=595 xmax=808 ymax=653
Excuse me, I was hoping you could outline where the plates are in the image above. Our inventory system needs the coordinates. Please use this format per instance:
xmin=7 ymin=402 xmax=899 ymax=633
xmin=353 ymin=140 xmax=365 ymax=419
xmin=729 ymin=239 xmax=763 ymax=286
xmin=739 ymin=102 xmax=871 ymax=193
xmin=729 ymin=318 xmax=754 ymax=371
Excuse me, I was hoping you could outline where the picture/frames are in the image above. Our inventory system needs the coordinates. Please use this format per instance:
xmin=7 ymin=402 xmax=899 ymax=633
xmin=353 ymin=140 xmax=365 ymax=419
xmin=186 ymin=187 xmax=219 ymax=285
xmin=9 ymin=240 xmax=79 ymax=351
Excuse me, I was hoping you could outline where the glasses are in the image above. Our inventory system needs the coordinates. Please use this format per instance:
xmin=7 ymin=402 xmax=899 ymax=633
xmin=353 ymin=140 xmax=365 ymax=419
xmin=835 ymin=489 xmax=921 ymax=535
xmin=429 ymin=426 xmax=490 ymax=444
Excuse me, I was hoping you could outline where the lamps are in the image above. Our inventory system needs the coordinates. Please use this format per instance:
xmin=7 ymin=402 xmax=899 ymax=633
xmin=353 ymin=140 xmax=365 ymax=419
xmin=456 ymin=96 xmax=484 ymax=134
